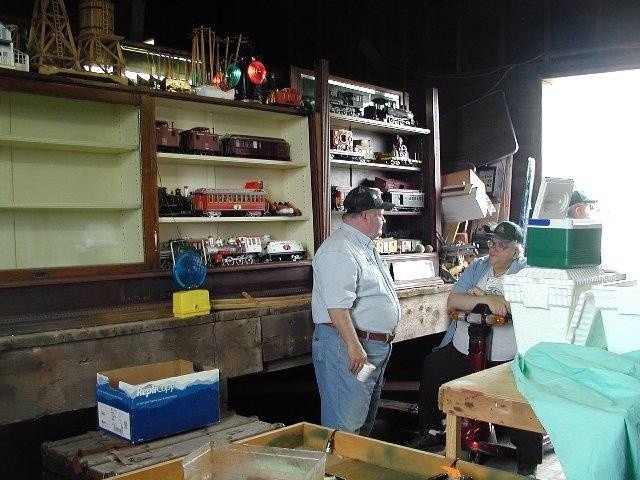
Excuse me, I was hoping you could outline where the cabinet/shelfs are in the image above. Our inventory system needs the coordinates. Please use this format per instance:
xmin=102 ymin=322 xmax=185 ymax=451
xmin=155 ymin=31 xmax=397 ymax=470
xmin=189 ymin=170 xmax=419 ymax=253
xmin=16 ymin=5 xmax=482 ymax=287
xmin=0 ymin=58 xmax=442 ymax=295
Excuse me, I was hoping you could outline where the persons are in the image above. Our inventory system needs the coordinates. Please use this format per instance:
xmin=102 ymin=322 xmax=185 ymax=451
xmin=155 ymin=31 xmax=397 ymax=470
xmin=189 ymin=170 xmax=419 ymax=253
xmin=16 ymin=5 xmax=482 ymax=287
xmin=309 ymin=185 xmax=403 ymax=436
xmin=399 ymin=221 xmax=543 ymax=480
xmin=567 ymin=190 xmax=598 ymax=219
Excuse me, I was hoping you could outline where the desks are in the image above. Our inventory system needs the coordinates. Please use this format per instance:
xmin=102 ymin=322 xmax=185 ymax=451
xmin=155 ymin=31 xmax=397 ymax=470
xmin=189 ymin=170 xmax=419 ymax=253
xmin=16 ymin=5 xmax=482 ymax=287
xmin=438 ymin=361 xmax=548 ymax=463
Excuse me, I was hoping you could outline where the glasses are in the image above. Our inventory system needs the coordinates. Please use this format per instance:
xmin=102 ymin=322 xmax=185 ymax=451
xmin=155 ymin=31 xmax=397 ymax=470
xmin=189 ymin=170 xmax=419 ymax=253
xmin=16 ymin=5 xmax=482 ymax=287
xmin=486 ymin=239 xmax=514 ymax=249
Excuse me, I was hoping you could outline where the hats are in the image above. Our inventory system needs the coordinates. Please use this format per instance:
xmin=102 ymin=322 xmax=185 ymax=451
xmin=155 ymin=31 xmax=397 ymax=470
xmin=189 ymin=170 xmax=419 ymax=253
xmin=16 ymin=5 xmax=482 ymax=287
xmin=483 ymin=221 xmax=525 ymax=244
xmin=343 ymin=184 xmax=397 ymax=211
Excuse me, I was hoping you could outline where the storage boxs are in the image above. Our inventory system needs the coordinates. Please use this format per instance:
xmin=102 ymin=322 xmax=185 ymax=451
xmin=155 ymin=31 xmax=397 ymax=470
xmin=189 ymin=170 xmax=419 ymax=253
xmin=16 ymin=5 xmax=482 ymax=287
xmin=95 ymin=358 xmax=224 ymax=444
xmin=500 ymin=217 xmax=638 ymax=354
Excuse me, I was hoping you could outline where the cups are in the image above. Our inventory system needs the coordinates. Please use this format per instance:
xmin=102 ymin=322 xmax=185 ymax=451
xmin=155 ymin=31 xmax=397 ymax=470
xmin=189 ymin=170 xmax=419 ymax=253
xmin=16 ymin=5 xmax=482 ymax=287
xmin=356 ymin=362 xmax=376 ymax=383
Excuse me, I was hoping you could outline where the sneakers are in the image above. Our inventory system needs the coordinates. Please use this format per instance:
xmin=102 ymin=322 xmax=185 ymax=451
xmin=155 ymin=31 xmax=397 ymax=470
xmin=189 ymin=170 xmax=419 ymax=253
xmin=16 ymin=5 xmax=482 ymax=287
xmin=400 ymin=429 xmax=446 ymax=451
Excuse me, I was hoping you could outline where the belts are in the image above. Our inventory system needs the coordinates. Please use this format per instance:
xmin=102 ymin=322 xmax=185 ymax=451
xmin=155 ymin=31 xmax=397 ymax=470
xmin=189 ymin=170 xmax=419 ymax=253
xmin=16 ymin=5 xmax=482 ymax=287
xmin=319 ymin=322 xmax=396 ymax=342
